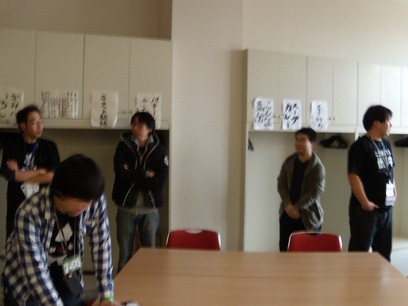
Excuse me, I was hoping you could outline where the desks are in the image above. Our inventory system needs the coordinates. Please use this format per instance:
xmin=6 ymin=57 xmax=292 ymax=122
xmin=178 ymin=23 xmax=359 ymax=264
xmin=92 ymin=247 xmax=408 ymax=306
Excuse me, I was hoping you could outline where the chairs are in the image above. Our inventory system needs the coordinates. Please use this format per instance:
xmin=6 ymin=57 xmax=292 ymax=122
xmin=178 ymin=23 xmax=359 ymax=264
xmin=165 ymin=228 xmax=221 ymax=251
xmin=287 ymin=231 xmax=342 ymax=252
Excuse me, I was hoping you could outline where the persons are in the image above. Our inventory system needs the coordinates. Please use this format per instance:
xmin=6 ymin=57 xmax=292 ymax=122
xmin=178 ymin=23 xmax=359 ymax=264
xmin=276 ymin=127 xmax=325 ymax=251
xmin=347 ymin=105 xmax=396 ymax=262
xmin=0 ymin=104 xmax=121 ymax=306
xmin=111 ymin=111 xmax=170 ymax=279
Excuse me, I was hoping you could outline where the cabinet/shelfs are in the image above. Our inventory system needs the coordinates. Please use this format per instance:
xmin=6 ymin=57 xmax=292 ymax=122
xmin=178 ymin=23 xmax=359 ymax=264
xmin=0 ymin=28 xmax=171 ymax=129
xmin=243 ymin=49 xmax=408 ymax=135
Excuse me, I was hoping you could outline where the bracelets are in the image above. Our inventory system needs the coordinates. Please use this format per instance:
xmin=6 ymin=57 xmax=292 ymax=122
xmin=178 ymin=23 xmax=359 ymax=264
xmin=101 ymin=297 xmax=114 ymax=302
